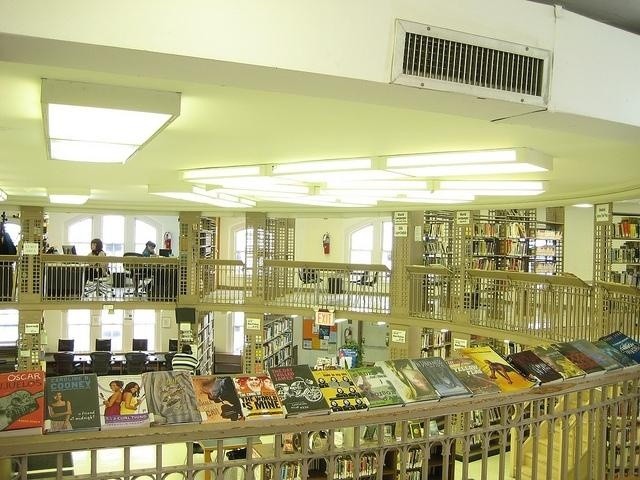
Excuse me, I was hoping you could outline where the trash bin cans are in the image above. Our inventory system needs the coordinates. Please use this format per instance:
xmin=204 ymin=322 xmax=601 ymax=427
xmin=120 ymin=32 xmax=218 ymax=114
xmin=112 ymin=273 xmax=126 ymax=288
xmin=328 ymin=278 xmax=342 ymax=294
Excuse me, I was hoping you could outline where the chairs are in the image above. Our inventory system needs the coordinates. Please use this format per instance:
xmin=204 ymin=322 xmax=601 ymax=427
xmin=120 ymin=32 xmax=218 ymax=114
xmin=53 ymin=353 xmax=74 ymax=376
xmin=124 ymin=252 xmax=147 ymax=297
xmin=124 ymin=353 xmax=150 ymax=375
xmin=90 ymin=352 xmax=110 ymax=375
xmin=182 ymin=442 xmax=216 ymax=480
xmin=84 ymin=266 xmax=115 ymax=300
xmin=165 ymin=352 xmax=177 ymax=370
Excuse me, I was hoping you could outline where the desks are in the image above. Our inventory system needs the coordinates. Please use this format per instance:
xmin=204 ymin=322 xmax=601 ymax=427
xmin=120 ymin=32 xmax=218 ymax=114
xmin=44 ymin=263 xmax=88 ymax=299
xmin=198 ymin=438 xmax=262 ymax=480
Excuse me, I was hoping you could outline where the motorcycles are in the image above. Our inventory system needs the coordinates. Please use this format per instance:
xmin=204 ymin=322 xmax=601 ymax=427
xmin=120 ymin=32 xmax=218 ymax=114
xmin=275 ymin=376 xmax=323 ymax=403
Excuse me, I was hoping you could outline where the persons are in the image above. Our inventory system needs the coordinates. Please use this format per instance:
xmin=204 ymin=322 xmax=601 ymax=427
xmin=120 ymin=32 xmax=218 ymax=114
xmin=246 ymin=376 xmax=263 ymax=393
xmin=318 ymin=375 xmax=368 ymax=412
xmin=143 ymin=240 xmax=158 ymax=293
xmin=102 ymin=380 xmax=124 ymax=416
xmin=119 ymin=382 xmax=142 ymax=414
xmin=484 ymin=359 xmax=514 ymax=385
xmin=261 ymin=377 xmax=274 ymax=390
xmin=86 ymin=238 xmax=109 ymax=284
xmin=47 ymin=388 xmax=72 ymax=431
xmin=171 ymin=344 xmax=201 ymax=375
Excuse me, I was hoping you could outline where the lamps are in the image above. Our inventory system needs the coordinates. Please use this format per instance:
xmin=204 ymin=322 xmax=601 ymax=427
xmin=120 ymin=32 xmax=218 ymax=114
xmin=45 ymin=186 xmax=91 ymax=206
xmin=42 ymin=77 xmax=182 ymax=168
xmin=147 ymin=144 xmax=554 ymax=209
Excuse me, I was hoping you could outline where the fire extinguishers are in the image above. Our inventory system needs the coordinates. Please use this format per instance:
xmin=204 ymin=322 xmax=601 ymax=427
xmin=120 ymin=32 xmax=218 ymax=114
xmin=163 ymin=232 xmax=171 ymax=250
xmin=344 ymin=327 xmax=351 ymax=345
xmin=323 ymin=232 xmax=331 ymax=255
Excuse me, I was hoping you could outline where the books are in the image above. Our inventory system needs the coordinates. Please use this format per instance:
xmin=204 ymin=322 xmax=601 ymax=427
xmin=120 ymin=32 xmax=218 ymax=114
xmin=413 ymin=357 xmax=474 ymax=401
xmin=421 ymin=329 xmax=525 ymax=359
xmin=190 ymin=374 xmax=246 ymax=426
xmin=142 ymin=369 xmax=203 ymax=429
xmin=43 ymin=373 xmax=102 ymax=437
xmin=443 ymin=357 xmax=502 ymax=397
xmin=460 ymin=345 xmax=539 ymax=395
xmin=312 ymin=369 xmax=369 ymax=417
xmin=198 ymin=312 xmax=294 ymax=371
xmin=506 ymin=349 xmax=564 ymax=387
xmin=551 ymin=342 xmax=607 ymax=378
xmin=568 ymin=338 xmax=625 ymax=374
xmin=268 ymin=363 xmax=332 ymax=419
xmin=272 ymin=399 xmax=555 ymax=480
xmin=603 ymin=384 xmax=639 ymax=480
xmin=233 ymin=373 xmax=286 ymax=421
xmin=375 ymin=357 xmax=441 ymax=406
xmin=346 ymin=366 xmax=406 ymax=413
xmin=599 ymin=330 xmax=640 ymax=365
xmin=423 ymin=210 xmax=640 ymax=309
xmin=97 ymin=372 xmax=150 ymax=435
xmin=591 ymin=340 xmax=638 ymax=368
xmin=0 ymin=369 xmax=46 ymax=438
xmin=532 ymin=343 xmax=586 ymax=380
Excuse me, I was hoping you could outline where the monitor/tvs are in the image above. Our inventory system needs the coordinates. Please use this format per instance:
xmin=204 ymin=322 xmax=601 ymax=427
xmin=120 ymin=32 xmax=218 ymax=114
xmin=175 ymin=308 xmax=195 ymax=323
xmin=96 ymin=339 xmax=111 ymax=351
xmin=169 ymin=340 xmax=178 ymax=351
xmin=59 ymin=340 xmax=74 ymax=351
xmin=159 ymin=249 xmax=172 ymax=257
xmin=62 ymin=246 xmax=76 ymax=254
xmin=133 ymin=339 xmax=147 ymax=351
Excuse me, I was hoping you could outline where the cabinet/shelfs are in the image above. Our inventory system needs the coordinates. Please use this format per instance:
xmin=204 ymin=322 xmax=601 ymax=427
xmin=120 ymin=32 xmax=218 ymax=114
xmin=177 ymin=211 xmax=221 ymax=301
xmin=593 ymin=204 xmax=640 ymax=289
xmin=243 ymin=212 xmax=295 ymax=305
xmin=18 ymin=209 xmax=46 ymax=302
xmin=252 ymin=398 xmax=557 ymax=480
xmin=178 ymin=312 xmax=293 ymax=375
xmin=421 ymin=330 xmax=523 ymax=359
xmin=422 ymin=207 xmax=568 ymax=315
xmin=17 ymin=311 xmax=45 ymax=371
